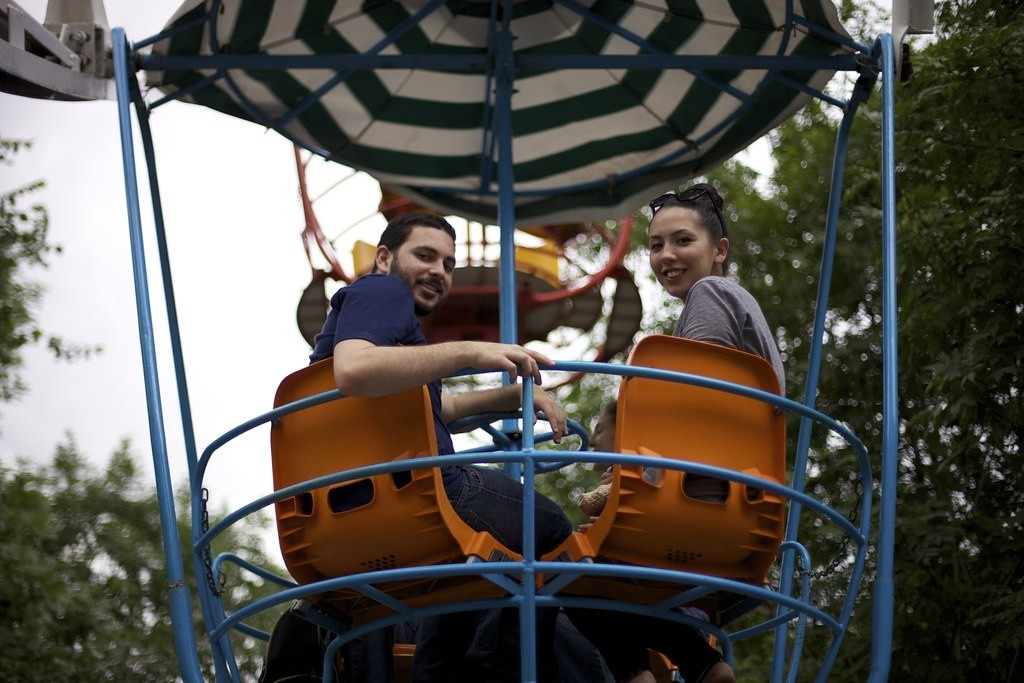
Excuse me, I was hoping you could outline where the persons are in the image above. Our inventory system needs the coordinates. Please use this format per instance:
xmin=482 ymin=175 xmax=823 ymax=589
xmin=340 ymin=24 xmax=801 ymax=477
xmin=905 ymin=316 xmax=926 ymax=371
xmin=582 ymin=180 xmax=789 ymax=683
xmin=308 ymin=208 xmax=572 ymax=683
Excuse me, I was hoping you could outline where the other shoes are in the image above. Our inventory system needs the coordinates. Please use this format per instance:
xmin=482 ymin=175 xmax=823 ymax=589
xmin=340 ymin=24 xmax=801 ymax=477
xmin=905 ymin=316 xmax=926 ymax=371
xmin=621 ymin=669 xmax=654 ymax=683
xmin=700 ymin=660 xmax=736 ymax=683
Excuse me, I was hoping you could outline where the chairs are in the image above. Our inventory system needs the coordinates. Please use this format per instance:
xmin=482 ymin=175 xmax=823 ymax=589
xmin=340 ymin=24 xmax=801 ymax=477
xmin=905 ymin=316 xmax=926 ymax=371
xmin=352 ymin=241 xmax=376 ymax=276
xmin=270 ymin=342 xmax=522 ymax=664
xmin=511 ymin=240 xmax=560 ymax=288
xmin=541 ymin=333 xmax=786 ymax=672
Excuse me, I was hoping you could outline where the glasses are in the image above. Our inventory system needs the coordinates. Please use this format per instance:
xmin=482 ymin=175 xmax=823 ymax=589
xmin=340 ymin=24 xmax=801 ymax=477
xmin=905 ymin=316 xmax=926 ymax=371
xmin=649 ymin=187 xmax=727 ymax=238
